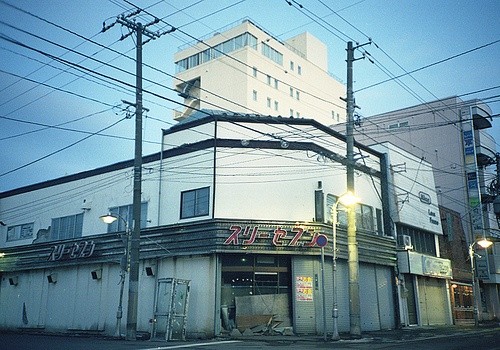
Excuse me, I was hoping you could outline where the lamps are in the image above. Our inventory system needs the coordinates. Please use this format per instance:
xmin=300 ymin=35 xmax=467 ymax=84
xmin=9 ymin=276 xmax=19 ymax=285
xmin=91 ymin=269 xmax=103 ymax=280
xmin=47 ymin=273 xmax=58 ymax=284
xmin=144 ymin=264 xmax=156 ymax=277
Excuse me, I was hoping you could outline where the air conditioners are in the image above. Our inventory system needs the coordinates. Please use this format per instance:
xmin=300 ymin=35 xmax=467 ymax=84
xmin=398 ymin=234 xmax=411 ymax=247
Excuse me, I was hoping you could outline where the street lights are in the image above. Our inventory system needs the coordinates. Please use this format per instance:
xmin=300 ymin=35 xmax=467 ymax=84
xmin=329 ymin=192 xmax=360 ymax=341
xmin=468 ymin=238 xmax=492 ymax=324
xmin=100 ymin=212 xmax=128 ymax=338
